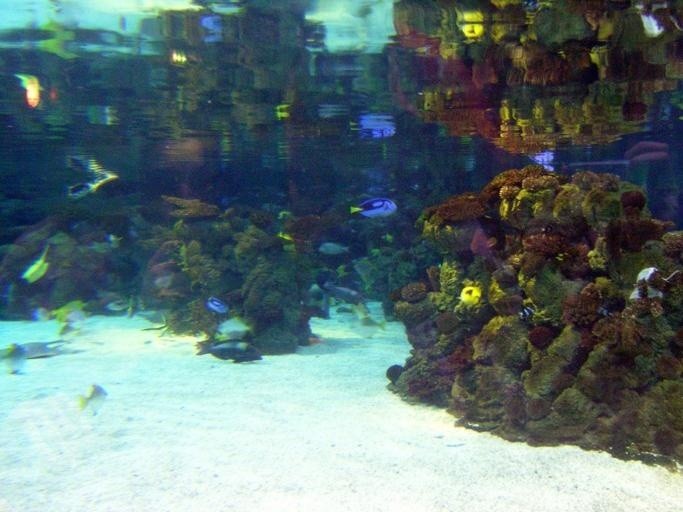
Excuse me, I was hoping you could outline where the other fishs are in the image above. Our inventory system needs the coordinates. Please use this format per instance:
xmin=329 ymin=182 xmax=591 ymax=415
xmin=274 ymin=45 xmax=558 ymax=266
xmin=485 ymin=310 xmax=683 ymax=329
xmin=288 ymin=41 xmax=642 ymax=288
xmin=0 ymin=140 xmax=683 ymax=375
